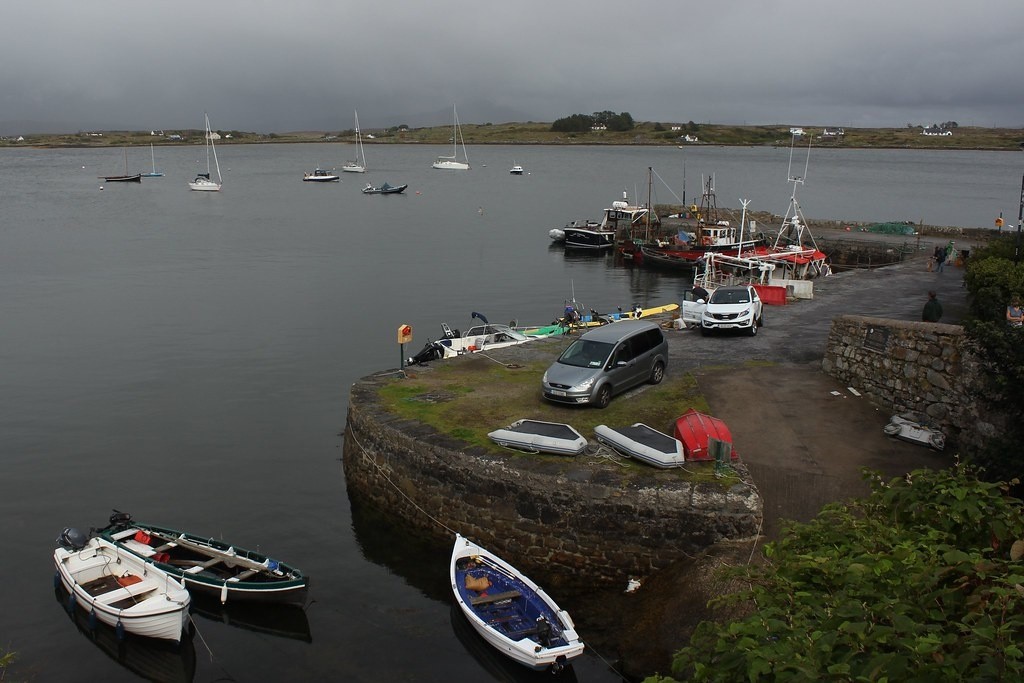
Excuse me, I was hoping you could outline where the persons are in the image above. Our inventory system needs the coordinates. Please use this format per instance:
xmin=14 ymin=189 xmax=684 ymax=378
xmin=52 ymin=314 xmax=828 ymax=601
xmin=922 ymin=289 xmax=943 ymax=321
xmin=1006 ymin=297 xmax=1024 ymax=327
xmin=935 ymin=246 xmax=948 ymax=272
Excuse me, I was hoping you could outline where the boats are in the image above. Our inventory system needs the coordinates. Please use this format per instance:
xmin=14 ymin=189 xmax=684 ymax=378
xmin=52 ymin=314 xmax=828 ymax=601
xmin=360 ymin=181 xmax=408 ymax=194
xmin=516 ymin=304 xmax=678 ymax=333
xmin=562 ymin=130 xmax=833 ymax=299
xmin=52 ymin=508 xmax=313 ymax=650
xmin=450 ymin=532 xmax=586 ymax=677
xmin=509 ymin=160 xmax=524 ymax=175
xmin=302 ymin=168 xmax=341 ymax=183
xmin=486 ymin=406 xmax=741 ymax=470
xmin=427 ymin=310 xmax=553 ymax=360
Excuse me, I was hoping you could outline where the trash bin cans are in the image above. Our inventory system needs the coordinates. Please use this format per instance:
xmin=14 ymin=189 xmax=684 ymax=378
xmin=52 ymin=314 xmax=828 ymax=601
xmin=961 ymin=249 xmax=969 ymax=259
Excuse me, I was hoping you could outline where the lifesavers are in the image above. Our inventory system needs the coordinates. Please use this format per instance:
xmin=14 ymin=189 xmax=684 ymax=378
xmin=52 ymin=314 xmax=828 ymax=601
xmin=703 ymin=237 xmax=712 ymax=246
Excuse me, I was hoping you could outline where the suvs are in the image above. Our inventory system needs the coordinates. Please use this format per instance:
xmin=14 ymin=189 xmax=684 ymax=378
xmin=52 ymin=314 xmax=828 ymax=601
xmin=541 ymin=319 xmax=669 ymax=409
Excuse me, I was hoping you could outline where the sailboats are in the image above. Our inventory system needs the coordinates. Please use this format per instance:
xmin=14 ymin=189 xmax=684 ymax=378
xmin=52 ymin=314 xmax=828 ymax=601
xmin=342 ymin=108 xmax=369 ymax=173
xmin=188 ymin=110 xmax=224 ymax=192
xmin=96 ymin=141 xmax=164 ymax=184
xmin=431 ymin=102 xmax=474 ymax=170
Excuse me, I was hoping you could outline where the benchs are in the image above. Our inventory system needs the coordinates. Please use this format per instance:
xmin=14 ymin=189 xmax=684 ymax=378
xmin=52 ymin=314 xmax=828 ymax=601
xmin=469 ymin=590 xmax=520 ymax=606
xmin=62 ymin=546 xmax=118 ymax=586
xmin=142 ymin=542 xmax=261 ymax=583
xmin=93 ymin=577 xmax=158 ymax=604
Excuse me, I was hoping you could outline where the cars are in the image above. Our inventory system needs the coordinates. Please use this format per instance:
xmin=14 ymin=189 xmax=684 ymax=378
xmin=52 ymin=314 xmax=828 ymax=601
xmin=682 ymin=285 xmax=764 ymax=337
